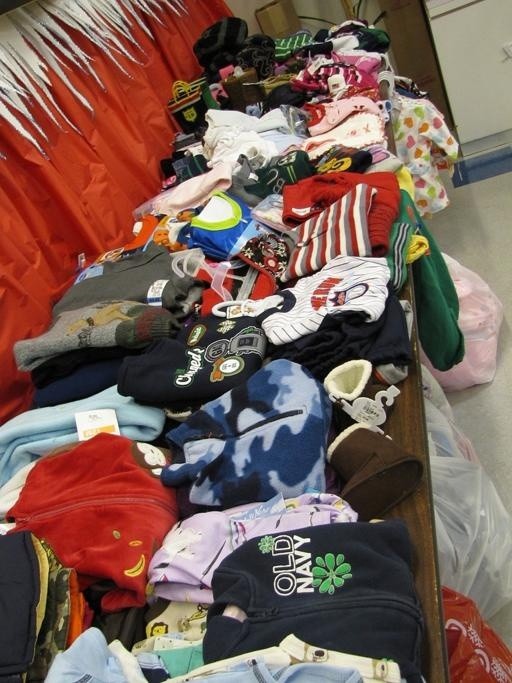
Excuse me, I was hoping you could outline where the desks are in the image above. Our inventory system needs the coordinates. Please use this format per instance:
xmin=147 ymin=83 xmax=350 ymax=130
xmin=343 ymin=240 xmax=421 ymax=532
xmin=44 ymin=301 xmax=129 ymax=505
xmin=0 ymin=23 xmax=451 ymax=682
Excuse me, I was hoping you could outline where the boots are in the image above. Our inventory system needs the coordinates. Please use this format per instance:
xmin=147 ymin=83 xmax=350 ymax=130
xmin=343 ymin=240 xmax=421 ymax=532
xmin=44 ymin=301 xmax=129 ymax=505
xmin=327 ymin=362 xmax=394 ymax=436
xmin=326 ymin=424 xmax=425 ymax=520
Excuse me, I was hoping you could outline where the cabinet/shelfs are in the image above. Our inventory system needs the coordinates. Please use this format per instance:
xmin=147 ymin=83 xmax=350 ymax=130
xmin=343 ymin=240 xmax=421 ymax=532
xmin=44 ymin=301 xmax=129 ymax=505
xmin=378 ymin=2 xmax=512 ymax=189
